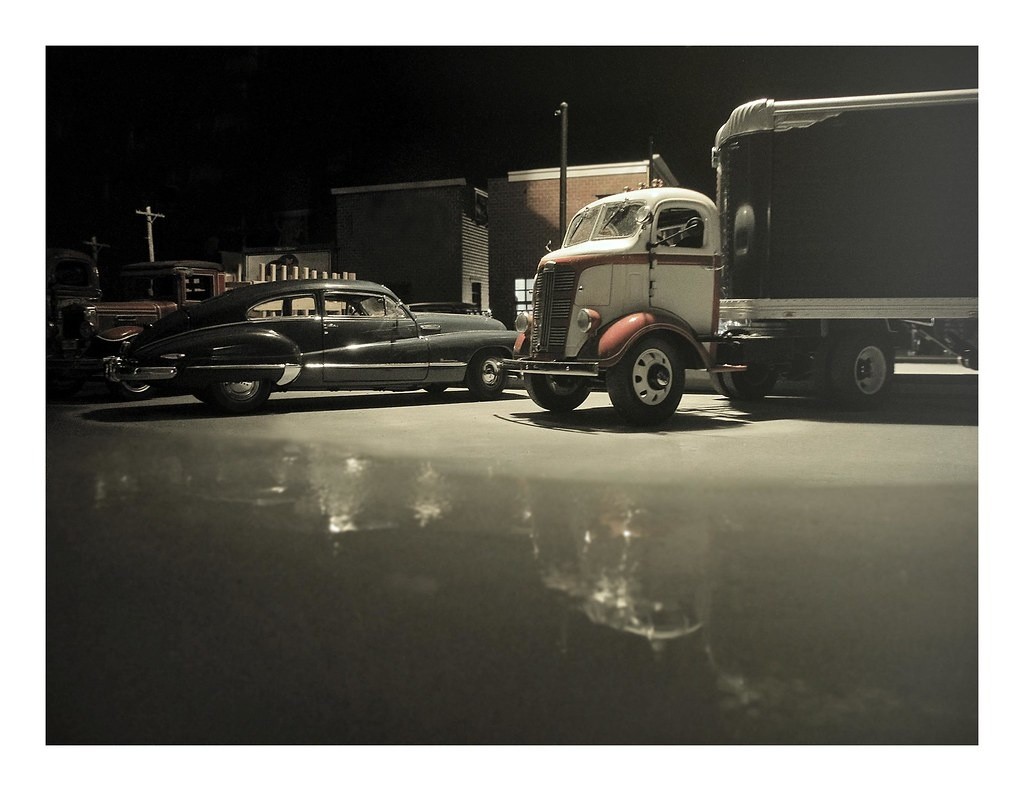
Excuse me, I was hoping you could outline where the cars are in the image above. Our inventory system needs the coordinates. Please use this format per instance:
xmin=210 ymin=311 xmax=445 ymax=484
xmin=101 ymin=278 xmax=517 ymax=418
xmin=46 ymin=246 xmax=226 ymax=401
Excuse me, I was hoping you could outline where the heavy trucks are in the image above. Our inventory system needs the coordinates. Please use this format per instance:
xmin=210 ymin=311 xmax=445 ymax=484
xmin=496 ymin=86 xmax=978 ymax=431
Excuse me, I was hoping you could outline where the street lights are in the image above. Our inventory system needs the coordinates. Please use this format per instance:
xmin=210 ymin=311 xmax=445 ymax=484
xmin=552 ymin=100 xmax=569 ymax=245
